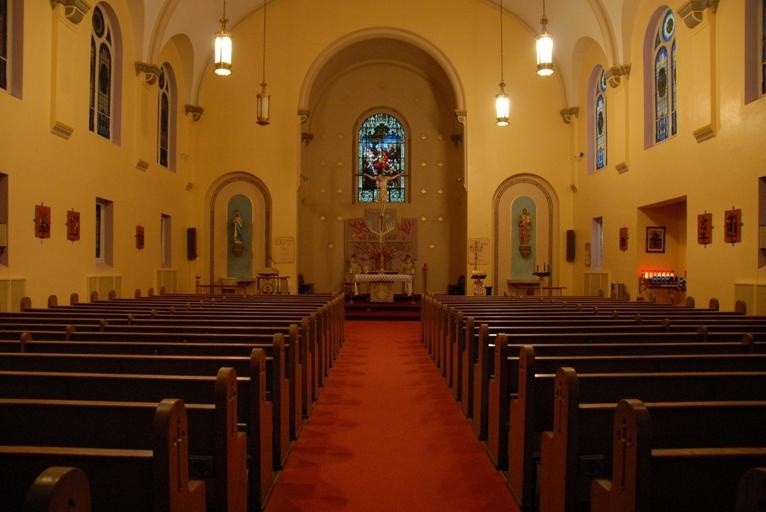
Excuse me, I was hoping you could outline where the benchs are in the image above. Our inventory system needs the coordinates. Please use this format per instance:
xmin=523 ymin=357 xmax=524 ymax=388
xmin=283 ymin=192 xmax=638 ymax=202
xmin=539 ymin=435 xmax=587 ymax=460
xmin=417 ymin=293 xmax=766 ymax=510
xmin=0 ymin=290 xmax=345 ymax=512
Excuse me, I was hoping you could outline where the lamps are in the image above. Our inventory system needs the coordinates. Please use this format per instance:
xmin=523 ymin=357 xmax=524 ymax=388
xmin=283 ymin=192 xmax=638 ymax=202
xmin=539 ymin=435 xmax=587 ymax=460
xmin=209 ymin=1 xmax=234 ymax=79
xmin=492 ymin=0 xmax=514 ymax=128
xmin=534 ymin=1 xmax=556 ymax=77
xmin=254 ymin=0 xmax=271 ymax=126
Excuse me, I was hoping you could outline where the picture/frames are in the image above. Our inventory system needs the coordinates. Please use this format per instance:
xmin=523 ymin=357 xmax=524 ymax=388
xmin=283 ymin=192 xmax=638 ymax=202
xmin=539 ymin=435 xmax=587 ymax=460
xmin=645 ymin=224 xmax=667 ymax=256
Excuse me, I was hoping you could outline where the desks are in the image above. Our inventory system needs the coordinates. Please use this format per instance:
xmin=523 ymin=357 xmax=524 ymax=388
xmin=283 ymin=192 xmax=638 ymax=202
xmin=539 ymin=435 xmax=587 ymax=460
xmin=353 ymin=274 xmax=413 ymax=297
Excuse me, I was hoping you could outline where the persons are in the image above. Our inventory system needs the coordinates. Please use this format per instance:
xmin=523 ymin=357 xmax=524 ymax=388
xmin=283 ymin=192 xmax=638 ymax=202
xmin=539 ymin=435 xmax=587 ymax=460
xmin=352 ymin=255 xmax=366 ymax=274
xmin=231 ymin=209 xmax=244 ymax=243
xmin=363 ymin=172 xmax=401 ymax=217
xmin=400 ymin=255 xmax=416 ymax=281
xmin=517 ymin=208 xmax=531 ymax=244
